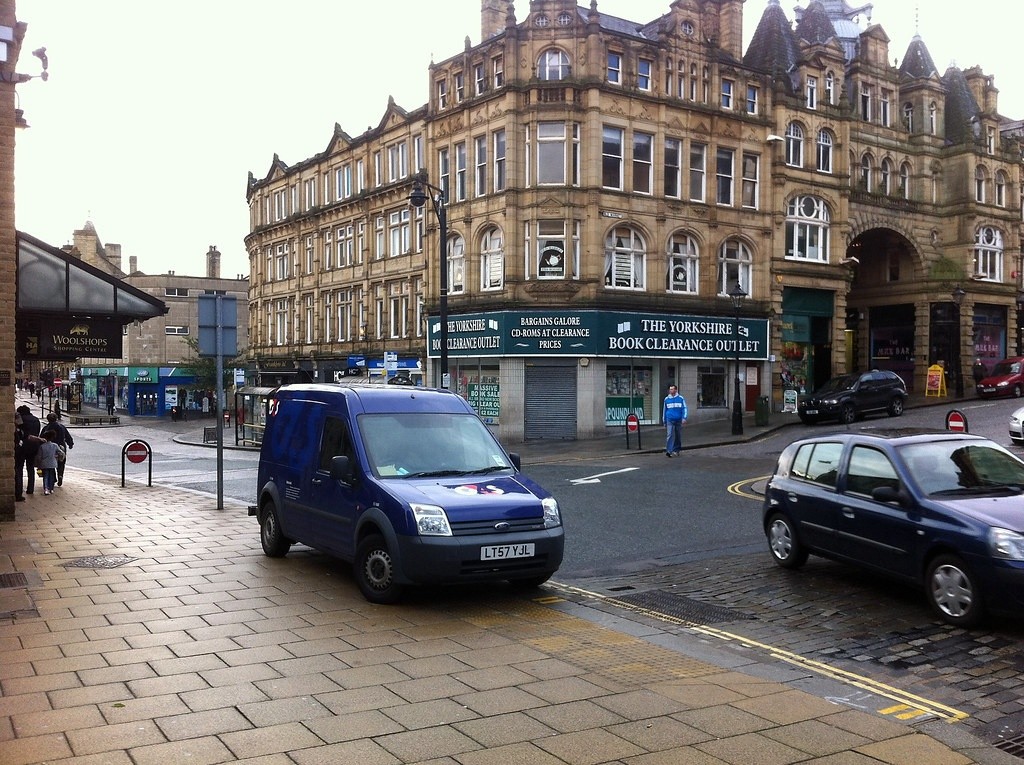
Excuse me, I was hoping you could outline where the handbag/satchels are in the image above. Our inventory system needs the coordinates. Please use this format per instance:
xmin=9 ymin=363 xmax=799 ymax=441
xmin=112 ymin=405 xmax=117 ymax=413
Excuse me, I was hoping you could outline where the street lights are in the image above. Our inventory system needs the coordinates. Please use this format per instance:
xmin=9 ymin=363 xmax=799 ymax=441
xmin=728 ymin=279 xmax=748 ymax=436
xmin=405 ymin=176 xmax=449 ymax=389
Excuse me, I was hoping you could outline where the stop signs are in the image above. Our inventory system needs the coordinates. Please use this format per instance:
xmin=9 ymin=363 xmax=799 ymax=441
xmin=627 ymin=415 xmax=637 ymax=430
xmin=126 ymin=443 xmax=148 ymax=463
xmin=224 ymin=413 xmax=229 ymax=419
xmin=948 ymin=412 xmax=965 ymax=431
xmin=54 ymin=378 xmax=62 ymax=387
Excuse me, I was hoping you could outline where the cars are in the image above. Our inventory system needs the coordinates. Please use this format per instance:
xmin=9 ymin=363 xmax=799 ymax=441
xmin=762 ymin=428 xmax=1024 ymax=626
xmin=977 ymin=356 xmax=1024 ymax=400
xmin=1008 ymin=406 xmax=1024 ymax=444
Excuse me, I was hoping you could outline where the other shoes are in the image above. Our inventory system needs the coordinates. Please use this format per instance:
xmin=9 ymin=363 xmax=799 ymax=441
xmin=51 ymin=489 xmax=54 ymax=494
xmin=16 ymin=497 xmax=26 ymax=502
xmin=44 ymin=489 xmax=50 ymax=495
xmin=58 ymin=476 xmax=62 ymax=486
xmin=666 ymin=451 xmax=672 ymax=457
xmin=25 ymin=490 xmax=34 ymax=494
xmin=672 ymin=451 xmax=681 ymax=457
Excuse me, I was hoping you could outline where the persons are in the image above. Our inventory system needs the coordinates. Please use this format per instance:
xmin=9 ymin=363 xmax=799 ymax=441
xmin=54 ymin=400 xmax=61 ymax=420
xmin=106 ymin=394 xmax=114 ymax=415
xmin=178 ymin=387 xmax=187 ymax=409
xmin=237 ymin=403 xmax=245 ymax=431
xmin=15 ymin=405 xmax=74 ymax=502
xmin=662 ymin=385 xmax=687 ymax=457
xmin=972 ymin=359 xmax=987 ymax=390
xmin=194 ymin=389 xmax=213 ymax=410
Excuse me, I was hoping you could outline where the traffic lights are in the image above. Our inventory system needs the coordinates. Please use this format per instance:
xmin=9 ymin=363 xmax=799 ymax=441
xmin=40 ymin=369 xmax=53 ymax=385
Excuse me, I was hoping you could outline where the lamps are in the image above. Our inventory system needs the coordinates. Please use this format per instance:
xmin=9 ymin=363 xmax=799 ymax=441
xmin=134 ymin=319 xmax=138 ymax=326
xmin=838 ymin=255 xmax=860 ymax=266
xmin=971 ymin=272 xmax=988 ymax=281
xmin=362 ymin=247 xmax=370 ymax=252
xmin=766 ymin=134 xmax=784 ymax=145
xmin=295 ymin=263 xmax=301 ymax=266
xmin=313 ymin=258 xmax=320 ymax=263
xmin=257 ymin=272 xmax=263 ymax=276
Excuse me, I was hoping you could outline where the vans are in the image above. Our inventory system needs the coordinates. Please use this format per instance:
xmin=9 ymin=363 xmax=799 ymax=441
xmin=247 ymin=382 xmax=566 ymax=605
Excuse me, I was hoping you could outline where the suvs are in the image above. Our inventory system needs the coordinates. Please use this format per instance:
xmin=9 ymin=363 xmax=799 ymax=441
xmin=798 ymin=371 xmax=908 ymax=426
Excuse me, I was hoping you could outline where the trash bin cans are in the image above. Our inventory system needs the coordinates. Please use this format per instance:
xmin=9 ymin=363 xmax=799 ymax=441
xmin=171 ymin=406 xmax=180 ymax=420
xmin=755 ymin=395 xmax=769 ymax=426
